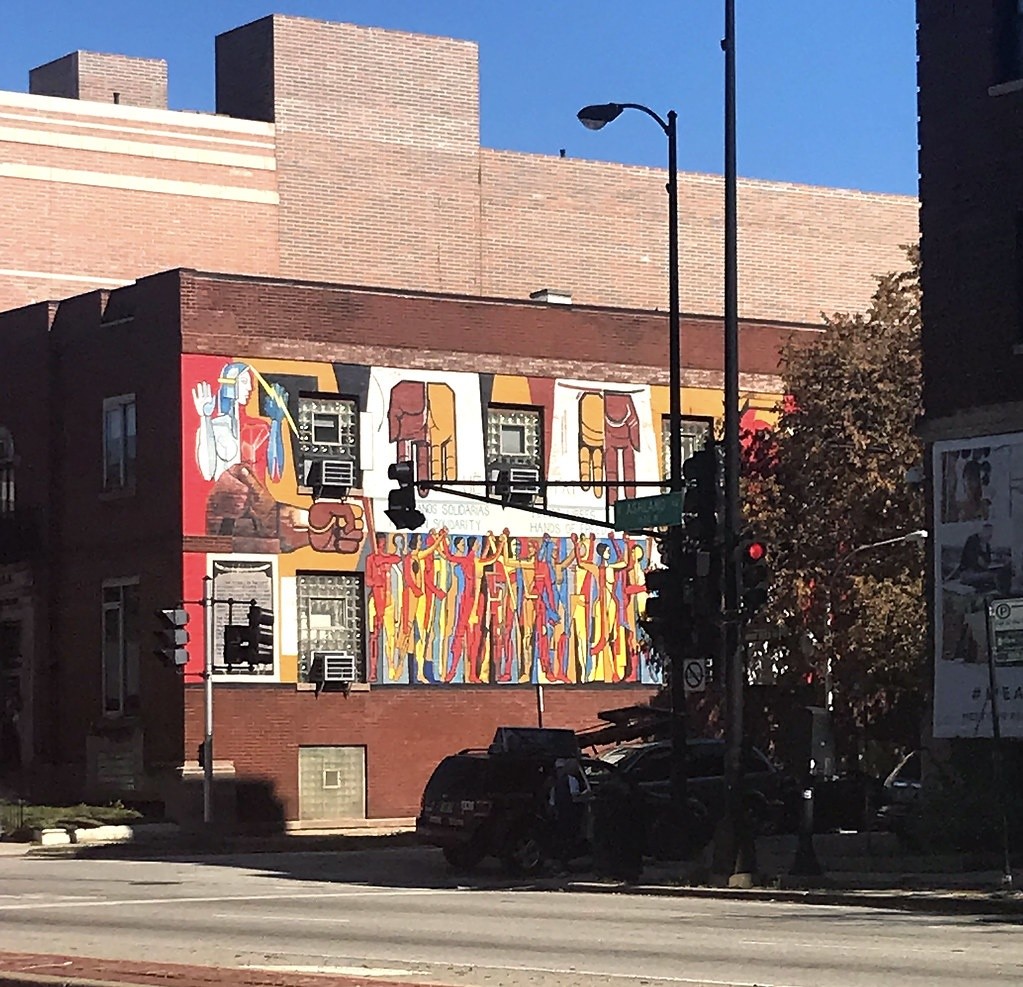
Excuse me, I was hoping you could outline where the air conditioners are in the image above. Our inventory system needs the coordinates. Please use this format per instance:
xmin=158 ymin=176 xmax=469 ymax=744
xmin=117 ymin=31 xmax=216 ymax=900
xmin=314 ymin=458 xmax=353 ymax=504
xmin=313 ymin=653 xmax=355 ymax=698
xmin=499 ymin=466 xmax=540 ymax=510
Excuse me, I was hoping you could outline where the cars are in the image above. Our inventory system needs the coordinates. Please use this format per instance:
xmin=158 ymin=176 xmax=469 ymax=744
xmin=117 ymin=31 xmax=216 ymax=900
xmin=416 ymin=727 xmax=920 ymax=876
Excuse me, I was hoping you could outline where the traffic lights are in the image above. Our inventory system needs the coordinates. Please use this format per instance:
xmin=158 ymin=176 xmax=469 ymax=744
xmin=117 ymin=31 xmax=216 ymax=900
xmin=639 ymin=567 xmax=675 ymax=645
xmin=383 ymin=460 xmax=426 ymax=531
xmin=683 ymin=450 xmax=718 ymax=540
xmin=740 ymin=541 xmax=768 ymax=609
xmin=153 ymin=609 xmax=190 ymax=667
xmin=224 ymin=606 xmax=274 ymax=665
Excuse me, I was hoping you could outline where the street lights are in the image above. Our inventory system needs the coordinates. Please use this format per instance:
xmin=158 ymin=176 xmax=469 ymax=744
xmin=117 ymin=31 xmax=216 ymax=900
xmin=576 ymin=102 xmax=688 ymax=798
xmin=827 ymin=530 xmax=929 ymax=710
